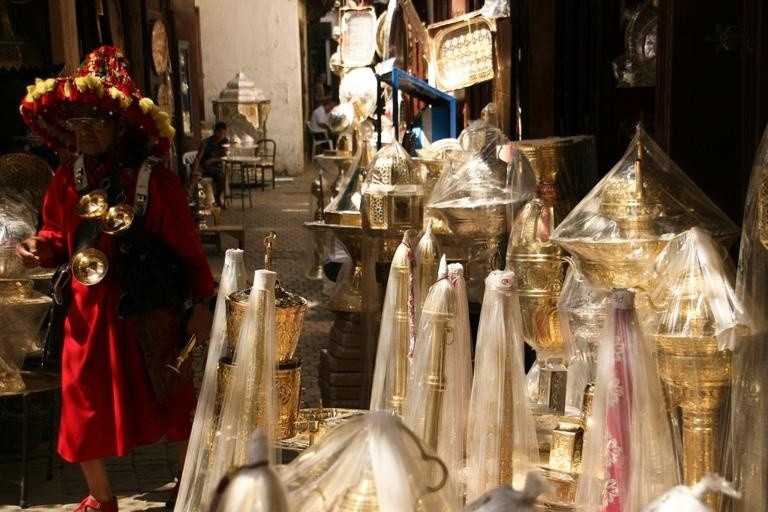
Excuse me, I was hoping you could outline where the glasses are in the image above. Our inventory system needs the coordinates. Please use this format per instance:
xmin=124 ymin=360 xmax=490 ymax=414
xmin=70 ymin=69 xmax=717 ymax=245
xmin=66 ymin=117 xmax=107 ymax=130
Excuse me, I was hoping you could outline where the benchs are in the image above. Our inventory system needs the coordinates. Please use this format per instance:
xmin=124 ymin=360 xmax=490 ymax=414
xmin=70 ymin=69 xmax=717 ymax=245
xmin=196 ymin=223 xmax=246 ymax=252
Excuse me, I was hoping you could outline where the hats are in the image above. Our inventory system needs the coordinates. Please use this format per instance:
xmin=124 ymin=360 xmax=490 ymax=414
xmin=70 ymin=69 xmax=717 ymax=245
xmin=16 ymin=43 xmax=176 ymax=160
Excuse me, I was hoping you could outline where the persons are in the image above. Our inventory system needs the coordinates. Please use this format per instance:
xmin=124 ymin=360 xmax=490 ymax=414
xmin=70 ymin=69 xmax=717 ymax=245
xmin=14 ymin=45 xmax=214 ymax=511
xmin=313 ymin=72 xmax=332 ymax=108
xmin=191 ymin=122 xmax=230 ymax=210
xmin=310 ymin=101 xmax=338 ymax=150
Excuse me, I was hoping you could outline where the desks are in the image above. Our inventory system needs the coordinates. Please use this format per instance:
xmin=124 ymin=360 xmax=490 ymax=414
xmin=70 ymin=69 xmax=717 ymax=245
xmin=220 ymin=156 xmax=262 ymax=210
xmin=267 ymin=406 xmax=381 ymax=454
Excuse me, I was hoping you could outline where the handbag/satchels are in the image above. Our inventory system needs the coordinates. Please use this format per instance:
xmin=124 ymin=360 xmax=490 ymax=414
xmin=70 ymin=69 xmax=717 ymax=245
xmin=20 ymin=263 xmax=72 ymax=378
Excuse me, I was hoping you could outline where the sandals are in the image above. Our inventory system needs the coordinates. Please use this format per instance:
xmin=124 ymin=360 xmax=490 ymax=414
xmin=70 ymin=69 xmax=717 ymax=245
xmin=74 ymin=493 xmax=118 ymax=512
xmin=169 ymin=488 xmax=177 ymax=501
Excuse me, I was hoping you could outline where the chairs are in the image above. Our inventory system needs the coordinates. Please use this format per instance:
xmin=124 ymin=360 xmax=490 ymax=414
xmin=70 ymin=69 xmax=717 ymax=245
xmin=244 ymin=140 xmax=278 ymax=193
xmin=181 ymin=151 xmax=199 ymax=181
xmin=0 ymin=261 xmax=75 ymax=512
xmin=305 ymin=120 xmax=334 ymax=161
